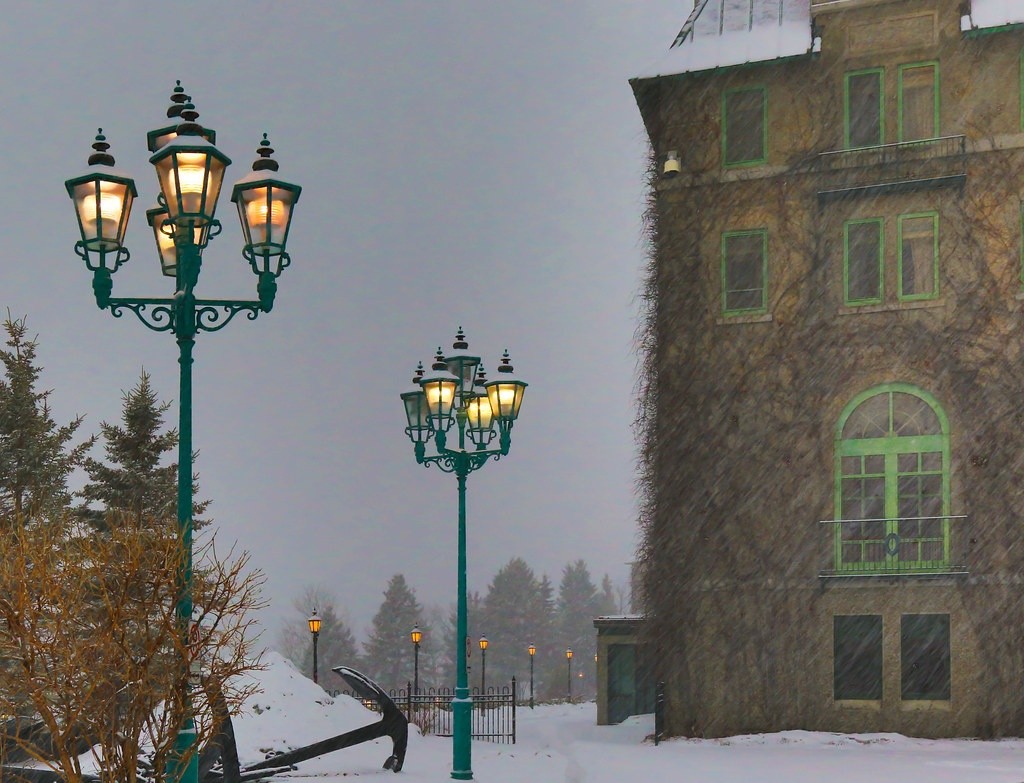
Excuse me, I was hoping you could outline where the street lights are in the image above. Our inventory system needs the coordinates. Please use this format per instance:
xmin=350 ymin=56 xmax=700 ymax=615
xmin=409 ymin=621 xmax=423 ymax=702
xmin=566 ymin=647 xmax=574 ymax=704
xmin=308 ymin=607 xmax=321 ymax=683
xmin=64 ymin=72 xmax=302 ymax=782
xmin=477 ymin=632 xmax=490 ymax=717
xmin=528 ymin=642 xmax=536 ymax=708
xmin=398 ymin=323 xmax=532 ymax=780
